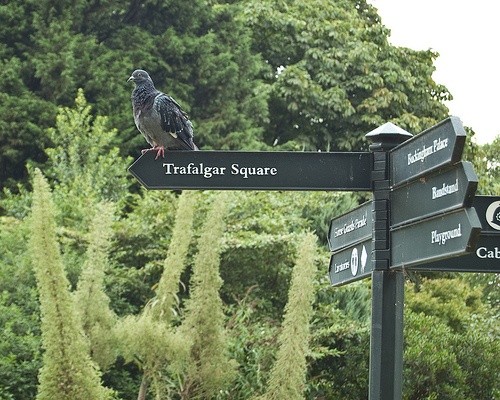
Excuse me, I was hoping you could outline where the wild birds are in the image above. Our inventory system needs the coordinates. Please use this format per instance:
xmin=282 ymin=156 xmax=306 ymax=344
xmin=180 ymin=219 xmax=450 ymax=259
xmin=127 ymin=71 xmax=195 ymax=160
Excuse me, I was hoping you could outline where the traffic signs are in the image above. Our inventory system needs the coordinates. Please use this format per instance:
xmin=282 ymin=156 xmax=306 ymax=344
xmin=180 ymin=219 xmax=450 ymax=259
xmin=127 ymin=115 xmax=500 ymax=288
